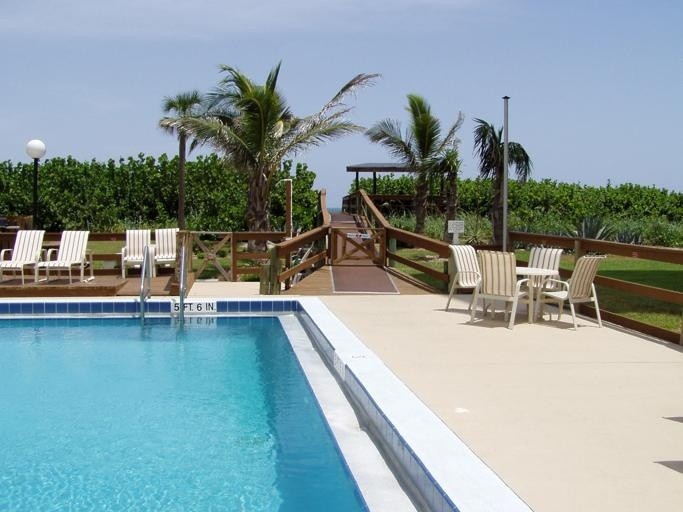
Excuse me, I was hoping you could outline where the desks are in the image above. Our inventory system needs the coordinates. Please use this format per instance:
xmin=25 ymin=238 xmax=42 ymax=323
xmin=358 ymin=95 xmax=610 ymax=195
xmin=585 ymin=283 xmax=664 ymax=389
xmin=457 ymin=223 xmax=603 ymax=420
xmin=503 ymin=266 xmax=559 ymax=322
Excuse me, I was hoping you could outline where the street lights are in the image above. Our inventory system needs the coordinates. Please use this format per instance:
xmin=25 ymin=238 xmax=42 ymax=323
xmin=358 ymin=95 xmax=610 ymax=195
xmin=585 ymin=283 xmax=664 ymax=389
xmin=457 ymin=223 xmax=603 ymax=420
xmin=25 ymin=139 xmax=46 ymax=230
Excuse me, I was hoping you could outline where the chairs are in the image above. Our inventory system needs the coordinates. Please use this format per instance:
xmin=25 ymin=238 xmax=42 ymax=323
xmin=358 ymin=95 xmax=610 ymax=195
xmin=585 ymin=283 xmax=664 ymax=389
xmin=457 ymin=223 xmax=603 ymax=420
xmin=0 ymin=230 xmax=45 ymax=286
xmin=121 ymin=229 xmax=152 ymax=279
xmin=152 ymin=228 xmax=179 ymax=278
xmin=522 ymin=248 xmax=563 ymax=320
xmin=469 ymin=249 xmax=532 ymax=330
xmin=32 ymin=230 xmax=94 ymax=284
xmin=444 ymin=245 xmax=486 ymax=316
xmin=533 ymin=255 xmax=606 ymax=331
